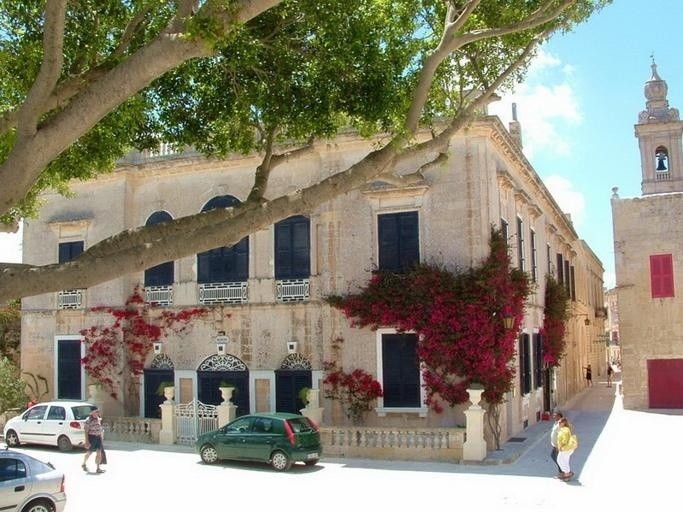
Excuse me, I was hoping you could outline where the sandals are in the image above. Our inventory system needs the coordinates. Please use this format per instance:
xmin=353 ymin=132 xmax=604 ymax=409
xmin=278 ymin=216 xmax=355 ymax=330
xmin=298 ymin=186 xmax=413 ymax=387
xmin=81 ymin=464 xmax=105 ymax=474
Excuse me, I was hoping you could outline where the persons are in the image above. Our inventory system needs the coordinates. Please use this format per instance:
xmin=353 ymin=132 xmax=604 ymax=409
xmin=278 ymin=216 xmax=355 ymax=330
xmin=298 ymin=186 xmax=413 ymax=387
xmin=555 ymin=416 xmax=577 ymax=482
xmin=605 ymin=360 xmax=616 ymax=388
xmin=80 ymin=405 xmax=105 ymax=473
xmin=581 ymin=364 xmax=593 ymax=388
xmin=549 ymin=410 xmax=563 ymax=479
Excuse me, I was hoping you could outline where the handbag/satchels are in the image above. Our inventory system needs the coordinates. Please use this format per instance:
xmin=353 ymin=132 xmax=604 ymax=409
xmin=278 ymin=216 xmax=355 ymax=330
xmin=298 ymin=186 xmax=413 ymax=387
xmin=94 ymin=444 xmax=108 ymax=464
xmin=557 ymin=434 xmax=577 ymax=453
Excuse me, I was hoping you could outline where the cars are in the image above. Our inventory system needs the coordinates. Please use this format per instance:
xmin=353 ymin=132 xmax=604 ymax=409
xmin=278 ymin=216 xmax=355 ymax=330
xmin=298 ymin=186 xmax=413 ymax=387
xmin=0 ymin=448 xmax=68 ymax=512
xmin=192 ymin=411 xmax=323 ymax=473
xmin=2 ymin=398 xmax=105 ymax=453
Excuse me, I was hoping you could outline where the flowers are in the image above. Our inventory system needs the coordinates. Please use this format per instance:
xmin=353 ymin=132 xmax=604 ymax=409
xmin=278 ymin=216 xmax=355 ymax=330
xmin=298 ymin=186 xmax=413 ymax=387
xmin=317 ymin=219 xmax=540 ymax=451
xmin=535 ymin=270 xmax=572 ymax=370
xmin=78 ymin=281 xmax=215 ymax=417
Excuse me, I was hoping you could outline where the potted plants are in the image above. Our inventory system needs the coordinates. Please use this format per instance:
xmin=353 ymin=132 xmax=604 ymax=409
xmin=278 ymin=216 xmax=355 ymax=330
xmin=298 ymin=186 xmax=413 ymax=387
xmin=155 ymin=381 xmax=176 ymax=403
xmin=217 ymin=380 xmax=239 ymax=404
xmin=297 ymin=387 xmax=319 ymax=408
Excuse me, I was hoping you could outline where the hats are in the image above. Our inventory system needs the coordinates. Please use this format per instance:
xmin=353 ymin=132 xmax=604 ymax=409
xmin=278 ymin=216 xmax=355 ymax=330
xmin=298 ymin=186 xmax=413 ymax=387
xmin=87 ymin=405 xmax=99 ymax=415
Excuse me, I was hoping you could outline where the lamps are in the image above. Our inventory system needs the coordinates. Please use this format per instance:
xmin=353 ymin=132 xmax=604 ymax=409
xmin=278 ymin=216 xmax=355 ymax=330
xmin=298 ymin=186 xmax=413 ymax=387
xmin=501 ymin=314 xmax=515 ymax=330
